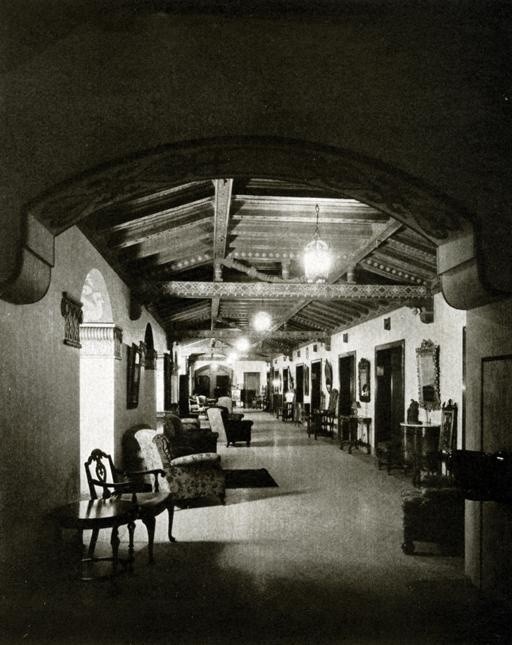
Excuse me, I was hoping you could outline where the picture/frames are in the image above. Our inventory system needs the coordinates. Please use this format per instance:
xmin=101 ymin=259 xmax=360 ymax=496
xmin=416 ymin=339 xmax=443 ymax=409
xmin=357 ymin=358 xmax=371 ymax=402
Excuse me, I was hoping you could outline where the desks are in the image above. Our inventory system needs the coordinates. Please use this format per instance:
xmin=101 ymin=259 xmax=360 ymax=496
xmin=258 ymin=384 xmax=444 ymax=490
xmin=39 ymin=498 xmax=139 ymax=575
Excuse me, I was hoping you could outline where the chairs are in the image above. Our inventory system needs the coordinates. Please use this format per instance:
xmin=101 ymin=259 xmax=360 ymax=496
xmin=85 ymin=395 xmax=253 ymax=567
xmin=400 ymin=469 xmax=465 ymax=556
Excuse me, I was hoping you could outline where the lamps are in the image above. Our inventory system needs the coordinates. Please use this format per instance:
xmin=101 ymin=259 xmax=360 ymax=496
xmin=298 ymin=207 xmax=336 ymax=286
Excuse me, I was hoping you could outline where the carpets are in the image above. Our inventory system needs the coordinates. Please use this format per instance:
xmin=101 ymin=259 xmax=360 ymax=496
xmin=222 ymin=466 xmax=278 ymax=489
